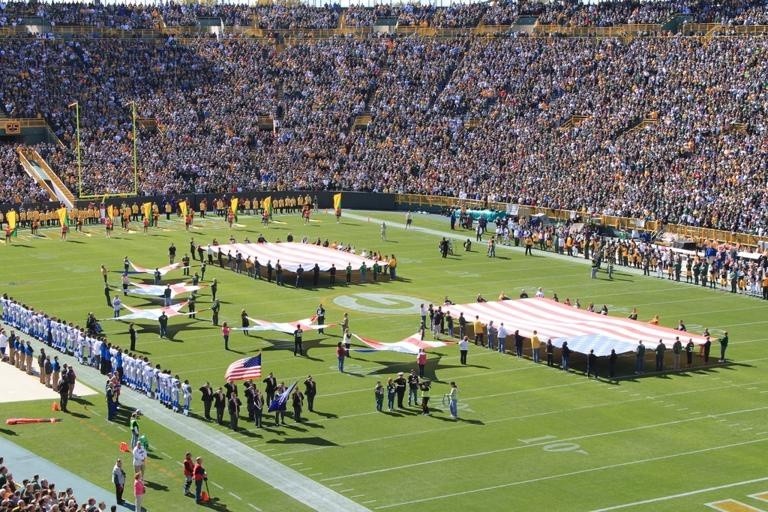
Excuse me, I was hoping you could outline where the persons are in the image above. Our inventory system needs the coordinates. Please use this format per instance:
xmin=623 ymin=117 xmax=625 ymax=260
xmin=170 ymin=369 xmax=459 ymax=428
xmin=1 ymin=0 xmax=766 ymax=379
xmin=0 ymin=380 xmax=211 ymax=512
